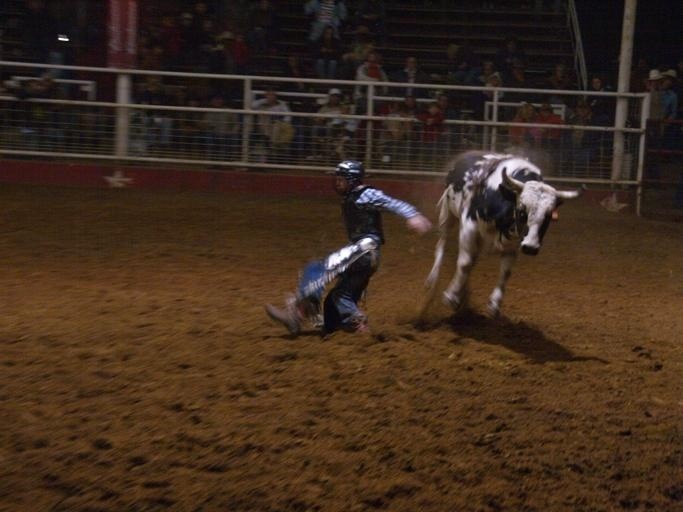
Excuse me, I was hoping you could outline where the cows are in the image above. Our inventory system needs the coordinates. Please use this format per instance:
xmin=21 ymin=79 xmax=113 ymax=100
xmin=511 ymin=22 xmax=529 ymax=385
xmin=422 ymin=149 xmax=588 ymax=319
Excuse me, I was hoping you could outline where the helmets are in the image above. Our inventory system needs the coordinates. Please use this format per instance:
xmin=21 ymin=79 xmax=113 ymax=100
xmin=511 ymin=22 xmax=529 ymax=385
xmin=334 ymin=160 xmax=363 ymax=187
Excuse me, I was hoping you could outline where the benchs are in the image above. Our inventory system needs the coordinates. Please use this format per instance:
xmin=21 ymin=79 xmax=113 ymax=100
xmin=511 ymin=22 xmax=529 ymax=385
xmin=0 ymin=1 xmax=590 ymax=123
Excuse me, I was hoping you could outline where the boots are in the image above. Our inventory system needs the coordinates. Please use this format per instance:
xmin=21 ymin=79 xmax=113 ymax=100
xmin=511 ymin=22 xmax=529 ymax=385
xmin=264 ymin=303 xmax=301 ymax=336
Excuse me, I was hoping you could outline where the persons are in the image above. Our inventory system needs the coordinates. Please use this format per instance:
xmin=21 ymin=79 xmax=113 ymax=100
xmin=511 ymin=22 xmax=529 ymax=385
xmin=263 ymin=161 xmax=433 ymax=334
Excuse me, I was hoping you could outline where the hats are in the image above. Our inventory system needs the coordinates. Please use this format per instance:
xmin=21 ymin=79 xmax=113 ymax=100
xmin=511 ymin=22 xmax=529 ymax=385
xmin=643 ymin=68 xmax=678 ymax=81
